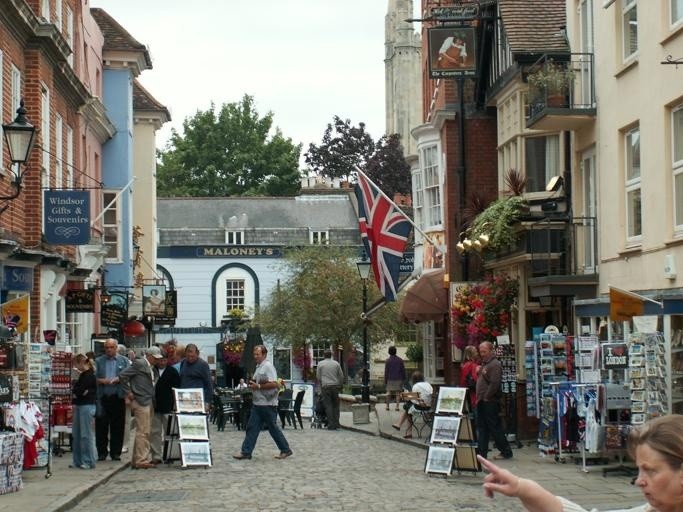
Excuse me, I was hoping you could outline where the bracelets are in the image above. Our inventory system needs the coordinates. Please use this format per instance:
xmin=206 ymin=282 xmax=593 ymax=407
xmin=258 ymin=385 xmax=261 ymax=390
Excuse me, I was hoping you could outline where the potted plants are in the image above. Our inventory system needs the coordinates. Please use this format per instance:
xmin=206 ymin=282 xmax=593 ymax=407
xmin=526 ymin=57 xmax=577 ymax=108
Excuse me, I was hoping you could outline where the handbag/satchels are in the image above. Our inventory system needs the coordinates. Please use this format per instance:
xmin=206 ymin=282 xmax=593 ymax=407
xmin=495 ymin=384 xmax=503 ymax=399
xmin=464 ymin=373 xmax=477 ymax=392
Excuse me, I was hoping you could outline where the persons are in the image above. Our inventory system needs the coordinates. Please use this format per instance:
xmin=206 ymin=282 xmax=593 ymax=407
xmin=461 ymin=347 xmax=480 ymax=443
xmin=277 ymin=377 xmax=286 ymax=395
xmin=233 ymin=345 xmax=293 ymax=460
xmin=72 ymin=338 xmax=248 ymax=470
xmin=316 ymin=350 xmax=344 ymax=429
xmin=478 ymin=412 xmax=683 ymax=510
xmin=148 ymin=289 xmax=164 ymax=311
xmin=384 ymin=346 xmax=405 ymax=410
xmin=392 ymin=372 xmax=433 ymax=438
xmin=476 ymin=341 xmax=513 ymax=466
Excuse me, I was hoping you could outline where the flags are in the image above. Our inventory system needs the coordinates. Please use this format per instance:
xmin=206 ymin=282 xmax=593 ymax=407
xmin=358 ymin=171 xmax=414 ymax=302
xmin=3 ymin=294 xmax=30 ymax=332
xmin=609 ymin=292 xmax=645 ymax=323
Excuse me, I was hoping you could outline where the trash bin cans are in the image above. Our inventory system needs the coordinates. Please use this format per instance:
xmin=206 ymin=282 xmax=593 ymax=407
xmin=352 ymin=384 xmax=371 ymax=412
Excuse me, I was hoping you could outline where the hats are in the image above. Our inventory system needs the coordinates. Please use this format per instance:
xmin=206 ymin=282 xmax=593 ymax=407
xmin=145 ymin=345 xmax=164 ymax=360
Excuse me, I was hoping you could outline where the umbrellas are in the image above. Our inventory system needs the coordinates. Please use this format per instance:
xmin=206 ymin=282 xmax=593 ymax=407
xmin=240 ymin=326 xmax=264 ymax=373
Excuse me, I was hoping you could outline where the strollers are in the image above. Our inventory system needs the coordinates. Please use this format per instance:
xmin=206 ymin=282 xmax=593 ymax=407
xmin=311 ymin=391 xmax=327 ymax=429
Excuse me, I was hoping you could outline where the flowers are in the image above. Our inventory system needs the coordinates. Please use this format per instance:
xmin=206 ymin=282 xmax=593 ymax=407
xmin=291 ymin=342 xmax=310 ymax=369
xmin=347 ymin=347 xmax=358 ymax=368
xmin=221 ymin=336 xmax=245 ymax=365
xmin=449 ymin=271 xmax=518 ymax=351
xmin=161 ymin=338 xmax=178 ymax=365
xmin=226 ymin=307 xmax=246 ymax=319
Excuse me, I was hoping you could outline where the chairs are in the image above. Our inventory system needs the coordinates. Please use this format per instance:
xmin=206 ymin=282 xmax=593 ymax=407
xmin=206 ymin=382 xmax=308 ymax=432
xmin=399 ymin=391 xmax=434 ymax=445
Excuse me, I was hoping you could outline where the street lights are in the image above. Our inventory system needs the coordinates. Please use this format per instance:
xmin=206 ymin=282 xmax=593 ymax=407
xmin=356 ymin=248 xmax=373 ymax=413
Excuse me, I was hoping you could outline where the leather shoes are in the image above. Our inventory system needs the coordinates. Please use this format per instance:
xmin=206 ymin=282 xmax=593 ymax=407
xmin=131 ymin=461 xmax=156 ymax=469
xmin=97 ymin=454 xmax=107 ymax=461
xmin=274 ymin=449 xmax=292 ymax=459
xmin=111 ymin=454 xmax=122 ymax=461
xmin=402 ymin=434 xmax=413 ymax=439
xmin=232 ymin=452 xmax=252 ymax=460
xmin=391 ymin=424 xmax=401 ymax=431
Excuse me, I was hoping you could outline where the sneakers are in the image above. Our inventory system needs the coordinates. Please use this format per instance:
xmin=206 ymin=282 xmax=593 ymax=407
xmin=150 ymin=459 xmax=162 ymax=465
xmin=493 ymin=452 xmax=512 ymax=460
xmin=68 ymin=463 xmax=80 ymax=468
xmin=79 ymin=463 xmax=96 ymax=470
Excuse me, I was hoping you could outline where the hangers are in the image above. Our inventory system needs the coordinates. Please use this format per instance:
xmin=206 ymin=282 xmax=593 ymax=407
xmin=556 ymin=382 xmax=597 ymax=405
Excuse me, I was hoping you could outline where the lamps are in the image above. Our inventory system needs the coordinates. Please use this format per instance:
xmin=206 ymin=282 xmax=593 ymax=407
xmin=455 ymin=220 xmax=495 ymax=255
xmin=93 ymin=279 xmax=111 ymax=305
xmin=0 ymin=96 xmax=40 ymax=217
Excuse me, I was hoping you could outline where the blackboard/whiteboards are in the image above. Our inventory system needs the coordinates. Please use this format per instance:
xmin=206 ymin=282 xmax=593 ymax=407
xmin=272 ymin=347 xmax=292 ymax=382
